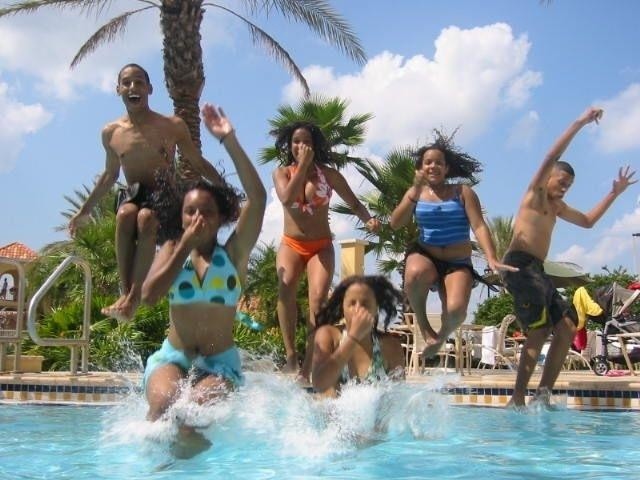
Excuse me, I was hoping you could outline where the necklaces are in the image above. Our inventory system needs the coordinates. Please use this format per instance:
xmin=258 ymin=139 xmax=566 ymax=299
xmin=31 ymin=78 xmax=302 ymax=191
xmin=427 ymin=182 xmax=446 ymax=196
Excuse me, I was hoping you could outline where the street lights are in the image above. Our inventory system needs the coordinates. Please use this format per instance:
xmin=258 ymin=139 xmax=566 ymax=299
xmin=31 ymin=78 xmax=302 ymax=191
xmin=632 ymin=231 xmax=640 ymax=276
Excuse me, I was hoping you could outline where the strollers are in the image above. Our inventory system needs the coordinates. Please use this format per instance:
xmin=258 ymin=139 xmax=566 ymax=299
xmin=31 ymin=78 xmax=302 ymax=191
xmin=573 ymin=280 xmax=640 ymax=377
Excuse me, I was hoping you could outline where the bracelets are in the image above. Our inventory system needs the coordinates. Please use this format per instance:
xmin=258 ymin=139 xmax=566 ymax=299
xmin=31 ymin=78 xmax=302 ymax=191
xmin=345 ymin=334 xmax=359 ymax=344
xmin=219 ymin=129 xmax=235 ymax=143
xmin=408 ymin=194 xmax=417 ymax=203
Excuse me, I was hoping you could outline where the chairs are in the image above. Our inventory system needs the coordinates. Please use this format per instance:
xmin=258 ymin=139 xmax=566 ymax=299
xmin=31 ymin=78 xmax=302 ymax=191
xmin=382 ymin=311 xmax=640 ymax=375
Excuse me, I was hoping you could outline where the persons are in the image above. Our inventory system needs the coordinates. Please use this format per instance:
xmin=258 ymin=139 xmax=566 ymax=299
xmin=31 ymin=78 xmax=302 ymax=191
xmin=139 ymin=104 xmax=267 ymax=459
xmin=272 ymin=122 xmax=383 ymax=384
xmin=0 ymin=277 xmax=8 ymax=300
xmin=497 ymin=107 xmax=640 ymax=405
xmin=68 ymin=63 xmax=228 ymax=323
xmin=308 ymin=275 xmax=407 ymax=403
xmin=389 ymin=122 xmax=520 ymax=359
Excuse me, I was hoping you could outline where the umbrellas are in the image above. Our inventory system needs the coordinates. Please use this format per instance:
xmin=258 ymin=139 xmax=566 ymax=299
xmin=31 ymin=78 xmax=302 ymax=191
xmin=480 ymin=262 xmax=595 ymax=287
